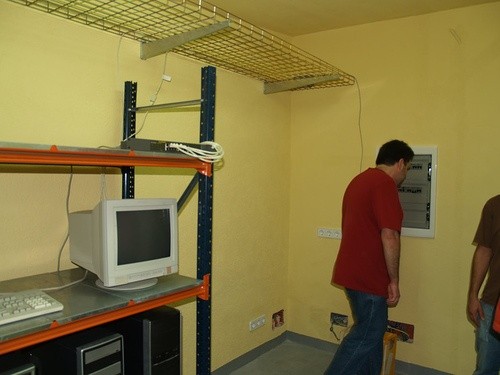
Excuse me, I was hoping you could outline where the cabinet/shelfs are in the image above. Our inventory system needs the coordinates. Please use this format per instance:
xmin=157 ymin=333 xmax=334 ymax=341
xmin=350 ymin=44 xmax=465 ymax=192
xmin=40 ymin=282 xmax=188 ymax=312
xmin=0 ymin=66 xmax=224 ymax=375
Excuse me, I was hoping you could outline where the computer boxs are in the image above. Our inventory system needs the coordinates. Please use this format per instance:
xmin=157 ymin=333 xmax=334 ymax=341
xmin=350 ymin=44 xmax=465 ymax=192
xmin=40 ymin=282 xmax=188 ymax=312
xmin=0 ymin=306 xmax=181 ymax=374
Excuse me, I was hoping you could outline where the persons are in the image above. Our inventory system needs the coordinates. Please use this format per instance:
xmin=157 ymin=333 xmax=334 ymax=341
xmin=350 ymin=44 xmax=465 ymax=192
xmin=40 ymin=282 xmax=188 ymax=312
xmin=466 ymin=193 xmax=500 ymax=375
xmin=325 ymin=139 xmax=414 ymax=375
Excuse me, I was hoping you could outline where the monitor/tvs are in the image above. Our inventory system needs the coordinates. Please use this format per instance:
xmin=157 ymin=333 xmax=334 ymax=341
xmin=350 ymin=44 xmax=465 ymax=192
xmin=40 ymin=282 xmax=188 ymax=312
xmin=68 ymin=198 xmax=178 ymax=291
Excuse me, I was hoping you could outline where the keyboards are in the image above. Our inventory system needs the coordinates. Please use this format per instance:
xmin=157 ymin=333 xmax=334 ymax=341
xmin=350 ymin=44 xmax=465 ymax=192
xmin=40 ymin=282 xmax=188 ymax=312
xmin=0 ymin=289 xmax=63 ymax=325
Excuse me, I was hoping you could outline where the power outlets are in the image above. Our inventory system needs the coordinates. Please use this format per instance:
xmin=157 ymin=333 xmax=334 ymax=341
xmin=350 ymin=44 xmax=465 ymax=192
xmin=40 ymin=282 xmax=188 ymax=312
xmin=317 ymin=225 xmax=341 ymax=241
